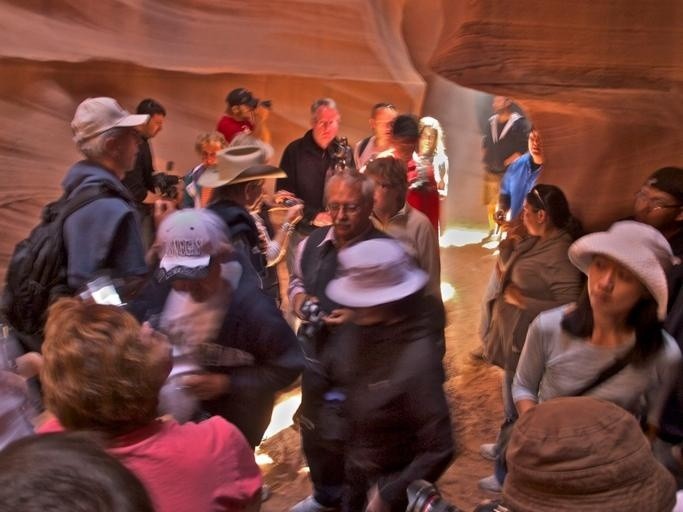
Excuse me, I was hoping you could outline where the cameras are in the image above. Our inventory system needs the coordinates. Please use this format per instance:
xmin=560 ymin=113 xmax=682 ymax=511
xmin=404 ymin=478 xmax=499 ymax=512
xmin=153 ymin=173 xmax=179 ymax=199
xmin=298 ymin=300 xmax=332 ymax=349
xmin=329 ymin=137 xmax=355 ymax=168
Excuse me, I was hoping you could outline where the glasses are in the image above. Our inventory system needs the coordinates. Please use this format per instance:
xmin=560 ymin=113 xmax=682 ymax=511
xmin=632 ymin=193 xmax=682 ymax=213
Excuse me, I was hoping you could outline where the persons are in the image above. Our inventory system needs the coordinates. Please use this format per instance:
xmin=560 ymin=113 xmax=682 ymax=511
xmin=275 ymin=98 xmax=356 ymax=273
xmin=325 ymin=236 xmax=459 ymax=512
xmin=197 ymin=143 xmax=305 ymax=306
xmin=631 ymin=164 xmax=683 ymax=255
xmin=182 ymin=130 xmax=230 ymax=212
xmin=20 ymin=96 xmax=177 ymax=299
xmin=356 ymin=155 xmax=442 ymax=335
xmin=473 ymin=183 xmax=584 ymax=494
xmin=416 ymin=116 xmax=449 ymax=238
xmin=375 ymin=113 xmax=439 ymax=246
xmin=511 ymin=220 xmax=681 ymax=451
xmin=37 ymin=296 xmax=264 ymax=509
xmin=465 ymin=128 xmax=544 ymax=359
xmin=125 ymin=98 xmax=178 ymax=218
xmin=287 ymin=167 xmax=409 ymax=510
xmin=480 ymin=93 xmax=533 ymax=246
xmin=1 ymin=432 xmax=152 ymax=512
xmin=216 ymin=88 xmax=273 ymax=144
xmin=353 ymin=104 xmax=401 ymax=173
xmin=128 ymin=207 xmax=305 ymax=453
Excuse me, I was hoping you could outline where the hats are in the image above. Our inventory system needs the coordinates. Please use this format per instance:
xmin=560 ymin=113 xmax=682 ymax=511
xmin=566 ymin=218 xmax=677 ymax=322
xmin=150 ymin=207 xmax=236 ymax=284
xmin=324 ymin=239 xmax=430 ymax=308
xmin=224 ymin=87 xmax=257 ymax=108
xmin=645 ymin=164 xmax=683 ymax=199
xmin=69 ymin=95 xmax=151 ymax=143
xmin=195 ymin=144 xmax=287 ymax=190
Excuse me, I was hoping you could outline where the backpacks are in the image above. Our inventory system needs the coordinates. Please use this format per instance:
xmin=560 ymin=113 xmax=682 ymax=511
xmin=3 ymin=177 xmax=126 ymax=348
